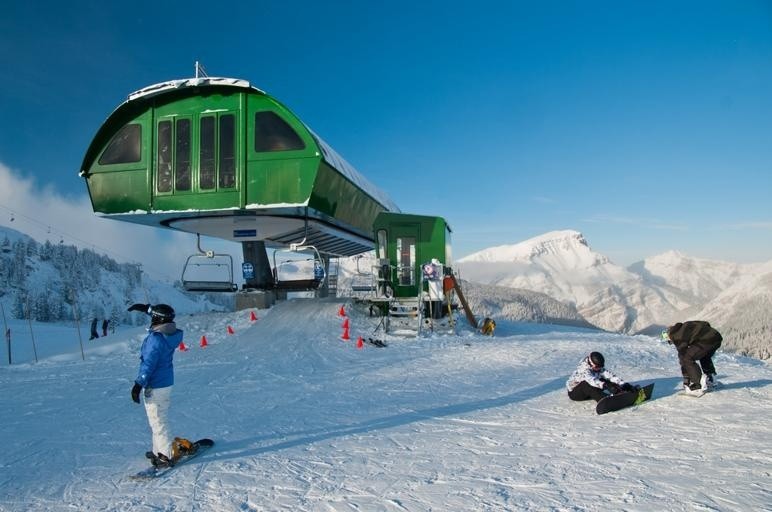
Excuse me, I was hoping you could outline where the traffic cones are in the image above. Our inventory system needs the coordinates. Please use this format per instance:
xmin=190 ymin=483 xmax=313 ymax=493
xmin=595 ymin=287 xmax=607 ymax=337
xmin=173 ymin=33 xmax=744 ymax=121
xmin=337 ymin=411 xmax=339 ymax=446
xmin=228 ymin=325 xmax=235 ymax=336
xmin=248 ymin=310 xmax=258 ymax=322
xmin=339 ymin=306 xmax=346 ymax=316
xmin=342 ymin=318 xmax=351 ymax=329
xmin=356 ymin=336 xmax=363 ymax=349
xmin=342 ymin=326 xmax=350 ymax=340
xmin=198 ymin=335 xmax=208 ymax=348
xmin=178 ymin=340 xmax=186 ymax=352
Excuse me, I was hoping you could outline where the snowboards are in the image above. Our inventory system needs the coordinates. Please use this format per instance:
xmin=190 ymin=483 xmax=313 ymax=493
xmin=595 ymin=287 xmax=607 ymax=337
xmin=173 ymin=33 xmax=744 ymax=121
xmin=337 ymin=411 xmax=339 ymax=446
xmin=679 ymin=388 xmax=711 ymax=398
xmin=451 ymin=269 xmax=480 ymax=329
xmin=596 ymin=383 xmax=655 ymax=414
xmin=131 ymin=439 xmax=214 ymax=482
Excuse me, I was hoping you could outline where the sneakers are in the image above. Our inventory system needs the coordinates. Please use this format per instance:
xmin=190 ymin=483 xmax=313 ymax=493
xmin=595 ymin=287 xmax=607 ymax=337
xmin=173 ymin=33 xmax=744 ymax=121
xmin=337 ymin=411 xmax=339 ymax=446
xmin=145 ymin=437 xmax=192 ymax=470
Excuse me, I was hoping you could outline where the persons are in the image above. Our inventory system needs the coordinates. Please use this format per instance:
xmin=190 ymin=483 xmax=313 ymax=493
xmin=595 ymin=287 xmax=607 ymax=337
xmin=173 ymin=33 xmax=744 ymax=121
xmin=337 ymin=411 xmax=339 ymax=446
xmin=100 ymin=319 xmax=110 ymax=337
xmin=565 ymin=351 xmax=634 ymax=405
xmin=126 ymin=302 xmax=184 ymax=468
xmin=378 ymin=264 xmax=397 ymax=299
xmin=661 ymin=319 xmax=724 ymax=398
xmin=89 ymin=318 xmax=100 ymax=341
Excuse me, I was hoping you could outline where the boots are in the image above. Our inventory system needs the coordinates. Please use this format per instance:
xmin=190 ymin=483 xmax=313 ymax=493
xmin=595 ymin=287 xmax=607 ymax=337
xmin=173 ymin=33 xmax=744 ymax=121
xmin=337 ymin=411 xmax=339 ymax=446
xmin=705 ymin=374 xmax=718 ymax=386
xmin=684 ymin=382 xmax=705 ymax=396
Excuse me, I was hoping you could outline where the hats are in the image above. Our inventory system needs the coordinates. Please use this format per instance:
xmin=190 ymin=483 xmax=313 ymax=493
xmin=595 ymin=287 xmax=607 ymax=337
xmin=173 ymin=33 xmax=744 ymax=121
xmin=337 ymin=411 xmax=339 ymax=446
xmin=589 ymin=351 xmax=605 ymax=368
xmin=660 ymin=327 xmax=671 ymax=344
xmin=152 ymin=303 xmax=175 ymax=319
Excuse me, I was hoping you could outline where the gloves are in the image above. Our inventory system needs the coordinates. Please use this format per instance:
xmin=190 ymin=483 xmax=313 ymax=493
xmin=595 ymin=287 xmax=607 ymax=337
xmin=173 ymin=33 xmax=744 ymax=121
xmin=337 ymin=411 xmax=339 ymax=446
xmin=131 ymin=381 xmax=142 ymax=404
xmin=127 ymin=304 xmax=152 ymax=315
